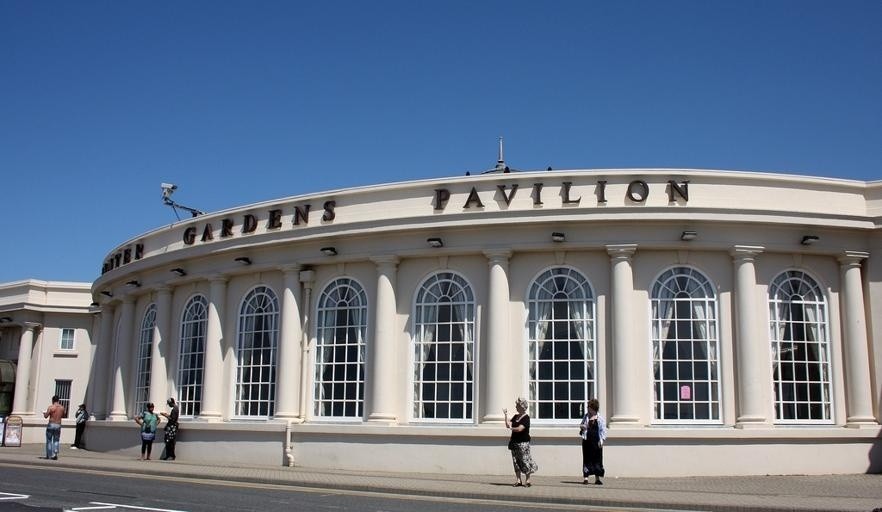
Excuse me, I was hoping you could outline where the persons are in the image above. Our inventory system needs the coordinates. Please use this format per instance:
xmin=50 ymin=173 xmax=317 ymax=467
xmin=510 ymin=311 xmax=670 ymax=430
xmin=135 ymin=402 xmax=162 ymax=460
xmin=43 ymin=395 xmax=67 ymax=460
xmin=160 ymin=397 xmax=179 ymax=460
xmin=71 ymin=403 xmax=89 ymax=447
xmin=501 ymin=396 xmax=538 ymax=488
xmin=578 ymin=398 xmax=608 ymax=485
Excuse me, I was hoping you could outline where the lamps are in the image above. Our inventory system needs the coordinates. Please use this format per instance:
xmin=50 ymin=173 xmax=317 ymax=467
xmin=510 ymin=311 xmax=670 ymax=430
xmin=801 ymin=236 xmax=819 ymax=245
xmin=427 ymin=238 xmax=443 ymax=247
xmin=235 ymin=257 xmax=251 ymax=265
xmin=321 ymin=247 xmax=337 ymax=255
xmin=552 ymin=232 xmax=565 ymax=242
xmin=91 ymin=268 xmax=186 ymax=308
xmin=681 ymin=231 xmax=697 ymax=240
xmin=0 ymin=317 xmax=11 ymax=323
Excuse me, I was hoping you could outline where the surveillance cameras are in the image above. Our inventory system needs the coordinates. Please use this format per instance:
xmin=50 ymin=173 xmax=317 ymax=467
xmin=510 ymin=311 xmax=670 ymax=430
xmin=159 ymin=181 xmax=178 ymax=191
